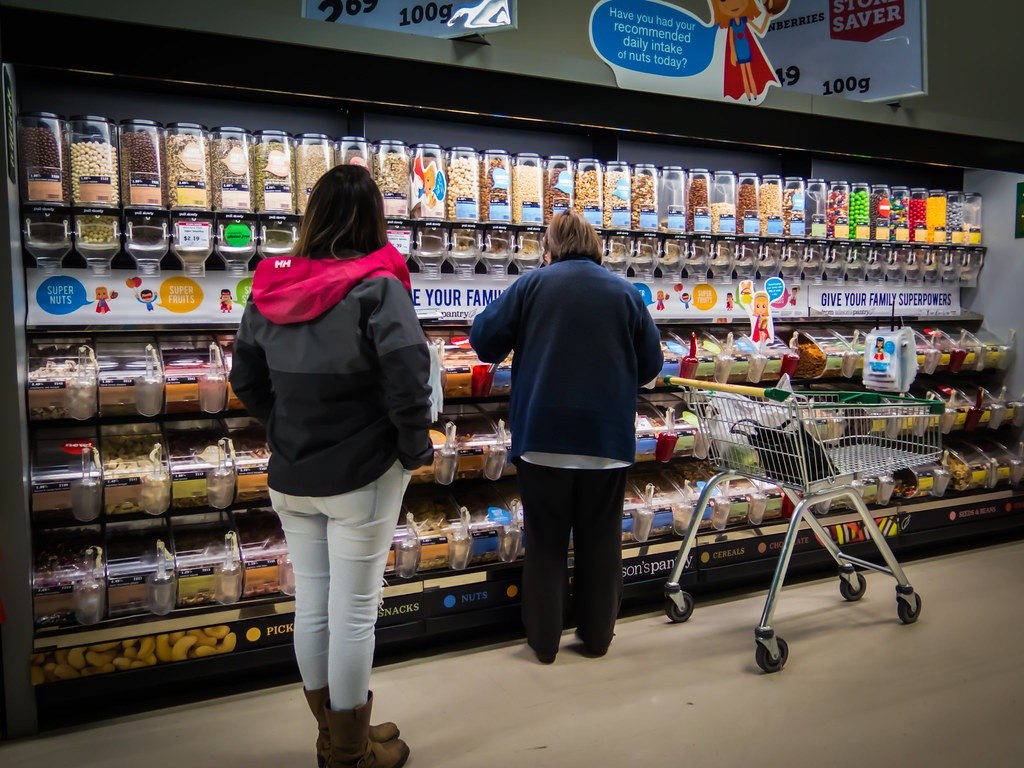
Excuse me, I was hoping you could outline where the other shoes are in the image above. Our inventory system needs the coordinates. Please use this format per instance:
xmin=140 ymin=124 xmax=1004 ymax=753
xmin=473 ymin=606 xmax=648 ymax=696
xmin=586 ymin=642 xmax=608 ymax=656
xmin=528 ymin=638 xmax=555 ymax=663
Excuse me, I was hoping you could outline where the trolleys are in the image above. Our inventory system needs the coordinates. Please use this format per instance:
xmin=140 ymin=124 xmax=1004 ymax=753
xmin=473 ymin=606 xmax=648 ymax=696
xmin=655 ymin=374 xmax=945 ymax=674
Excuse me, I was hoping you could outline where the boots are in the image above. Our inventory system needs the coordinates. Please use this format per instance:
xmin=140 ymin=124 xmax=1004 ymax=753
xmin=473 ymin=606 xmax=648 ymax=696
xmin=323 ymin=689 xmax=410 ymax=768
xmin=303 ymin=685 xmax=401 ymax=768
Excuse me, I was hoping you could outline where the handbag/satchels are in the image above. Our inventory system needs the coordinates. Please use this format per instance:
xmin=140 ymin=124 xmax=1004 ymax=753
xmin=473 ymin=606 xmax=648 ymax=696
xmin=706 ymin=371 xmax=802 ymax=472
xmin=730 ymin=419 xmax=841 ymax=486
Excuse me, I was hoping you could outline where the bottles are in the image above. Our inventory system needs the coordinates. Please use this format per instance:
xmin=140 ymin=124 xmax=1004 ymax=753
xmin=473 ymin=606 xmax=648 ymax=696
xmin=16 ymin=113 xmax=982 ymax=286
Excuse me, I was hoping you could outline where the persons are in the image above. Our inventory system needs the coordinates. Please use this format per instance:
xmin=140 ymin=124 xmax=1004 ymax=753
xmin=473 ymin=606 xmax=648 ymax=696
xmin=229 ymin=166 xmax=436 ymax=768
xmin=469 ymin=211 xmax=663 ymax=664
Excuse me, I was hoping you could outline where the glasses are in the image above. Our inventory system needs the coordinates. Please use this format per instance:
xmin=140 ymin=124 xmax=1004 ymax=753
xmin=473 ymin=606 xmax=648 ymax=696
xmin=543 ymin=249 xmax=548 ymax=262
